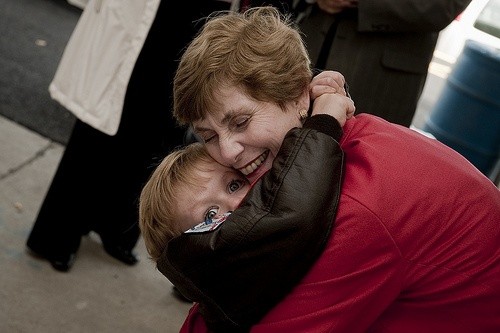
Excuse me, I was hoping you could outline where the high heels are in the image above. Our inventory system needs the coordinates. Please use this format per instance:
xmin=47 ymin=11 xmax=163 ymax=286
xmin=82 ymin=226 xmax=137 ymax=265
xmin=49 ymin=254 xmax=75 ymax=273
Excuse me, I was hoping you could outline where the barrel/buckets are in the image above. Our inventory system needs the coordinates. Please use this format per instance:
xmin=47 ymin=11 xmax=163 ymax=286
xmin=421 ymin=39 xmax=499 ymax=177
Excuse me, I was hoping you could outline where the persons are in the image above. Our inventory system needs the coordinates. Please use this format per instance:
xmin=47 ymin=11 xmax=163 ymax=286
xmin=285 ymin=0 xmax=472 ymax=128
xmin=138 ymin=67 xmax=356 ymax=333
xmin=27 ymin=0 xmax=240 ymax=272
xmin=173 ymin=7 xmax=500 ymax=333
